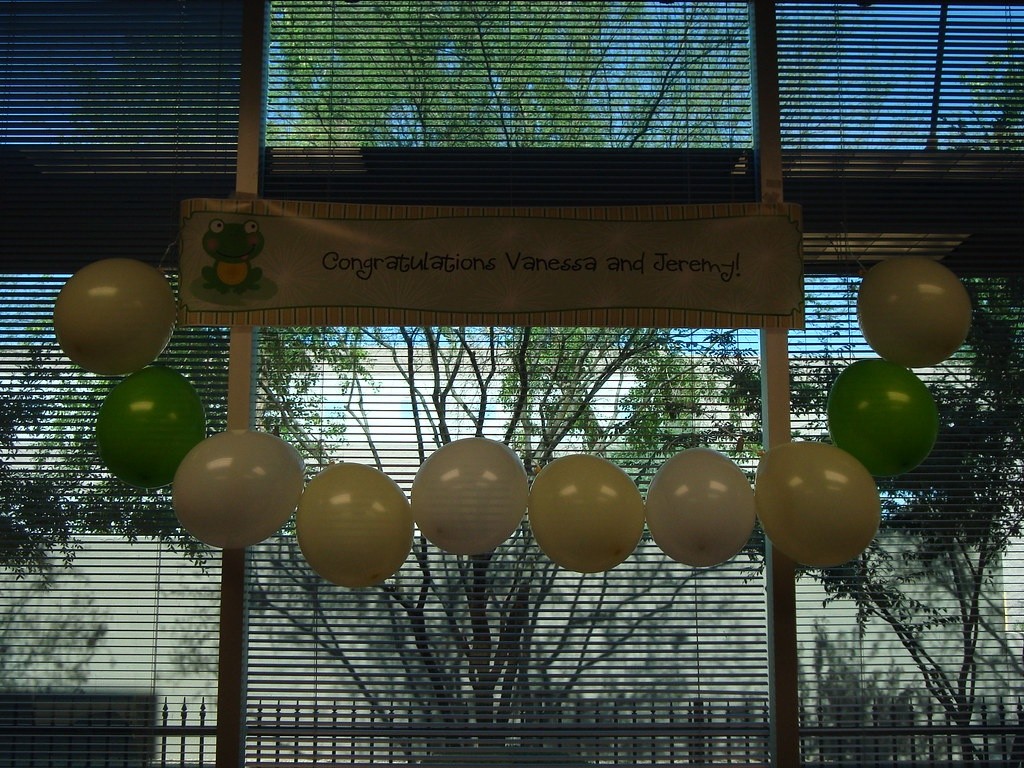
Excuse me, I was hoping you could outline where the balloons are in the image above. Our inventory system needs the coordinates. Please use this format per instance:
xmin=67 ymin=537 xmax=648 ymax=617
xmin=296 ymin=463 xmax=415 ymax=587
xmin=528 ymin=454 xmax=645 ymax=573
xmin=53 ymin=258 xmax=175 ymax=375
xmin=858 ymin=255 xmax=972 ymax=366
xmin=172 ymin=428 xmax=306 ymax=549
xmin=410 ymin=438 xmax=528 ymax=554
xmin=647 ymin=448 xmax=757 ymax=567
xmin=97 ymin=364 xmax=207 ymax=489
xmin=755 ymin=443 xmax=882 ymax=569
xmin=827 ymin=360 xmax=939 ymax=477
